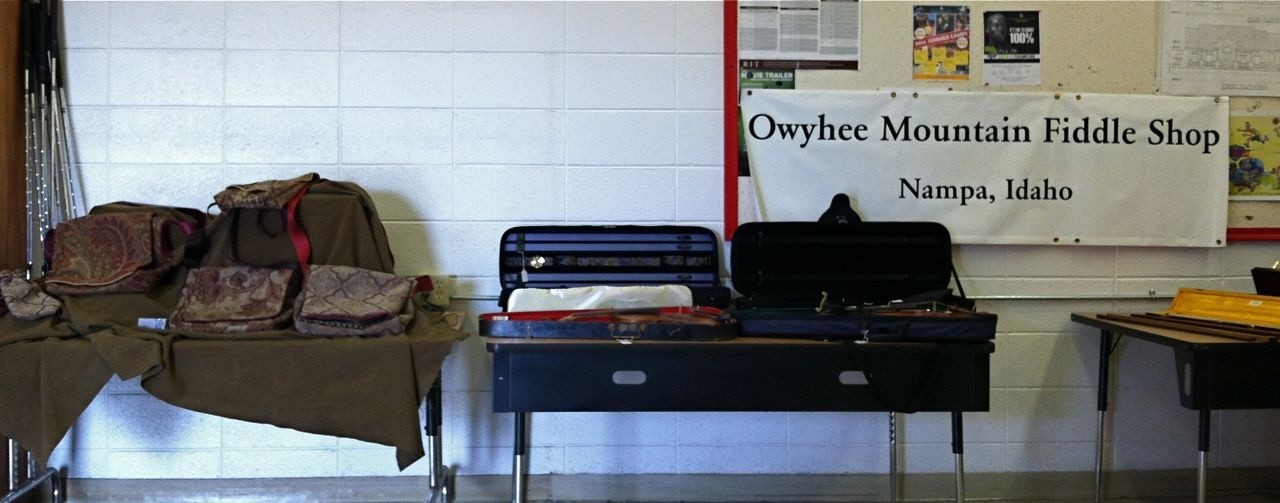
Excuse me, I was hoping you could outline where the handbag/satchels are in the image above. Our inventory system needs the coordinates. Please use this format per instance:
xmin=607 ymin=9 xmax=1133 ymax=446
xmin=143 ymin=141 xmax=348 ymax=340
xmin=45 ymin=210 xmax=175 ymax=294
xmin=293 ymin=263 xmax=433 ymax=338
xmin=214 ymin=172 xmax=320 ymax=210
xmin=170 ymin=266 xmax=294 ymax=332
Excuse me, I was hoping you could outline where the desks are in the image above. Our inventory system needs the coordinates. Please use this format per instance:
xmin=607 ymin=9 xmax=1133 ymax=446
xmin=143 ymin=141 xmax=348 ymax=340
xmin=0 ymin=332 xmax=460 ymax=503
xmin=486 ymin=338 xmax=995 ymax=503
xmin=1070 ymin=311 xmax=1280 ymax=503
